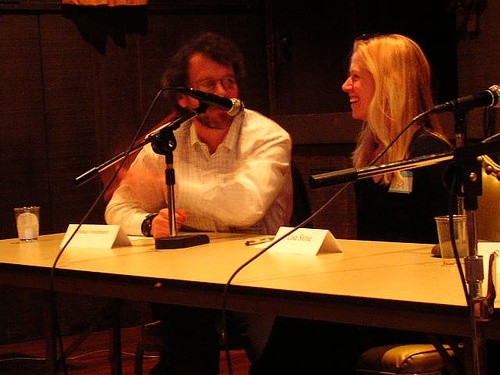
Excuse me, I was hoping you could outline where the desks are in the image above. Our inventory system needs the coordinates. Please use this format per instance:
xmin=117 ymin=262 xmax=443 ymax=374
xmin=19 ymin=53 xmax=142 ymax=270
xmin=0 ymin=232 xmax=500 ymax=375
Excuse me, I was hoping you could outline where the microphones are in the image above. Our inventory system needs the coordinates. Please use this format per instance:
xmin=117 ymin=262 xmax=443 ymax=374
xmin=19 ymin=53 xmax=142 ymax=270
xmin=173 ymin=86 xmax=244 ymax=116
xmin=431 ymin=85 xmax=500 ymax=114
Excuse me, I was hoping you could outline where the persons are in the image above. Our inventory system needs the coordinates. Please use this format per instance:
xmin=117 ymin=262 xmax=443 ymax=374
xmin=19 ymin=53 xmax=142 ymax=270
xmin=247 ymin=33 xmax=457 ymax=374
xmin=104 ymin=31 xmax=293 ymax=374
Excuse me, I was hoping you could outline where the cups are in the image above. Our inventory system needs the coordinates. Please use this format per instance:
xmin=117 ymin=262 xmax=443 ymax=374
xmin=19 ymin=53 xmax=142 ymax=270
xmin=14 ymin=206 xmax=41 ymax=240
xmin=434 ymin=215 xmax=471 ymax=265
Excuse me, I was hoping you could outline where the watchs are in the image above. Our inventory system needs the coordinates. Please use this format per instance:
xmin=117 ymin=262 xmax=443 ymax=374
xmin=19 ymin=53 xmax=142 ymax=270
xmin=141 ymin=215 xmax=156 ymax=237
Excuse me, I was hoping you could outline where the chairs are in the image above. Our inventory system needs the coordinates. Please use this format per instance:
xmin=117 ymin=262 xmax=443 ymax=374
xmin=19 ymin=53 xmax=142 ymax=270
xmin=355 ymin=154 xmax=500 ymax=375
xmin=135 ymin=160 xmax=311 ymax=375
